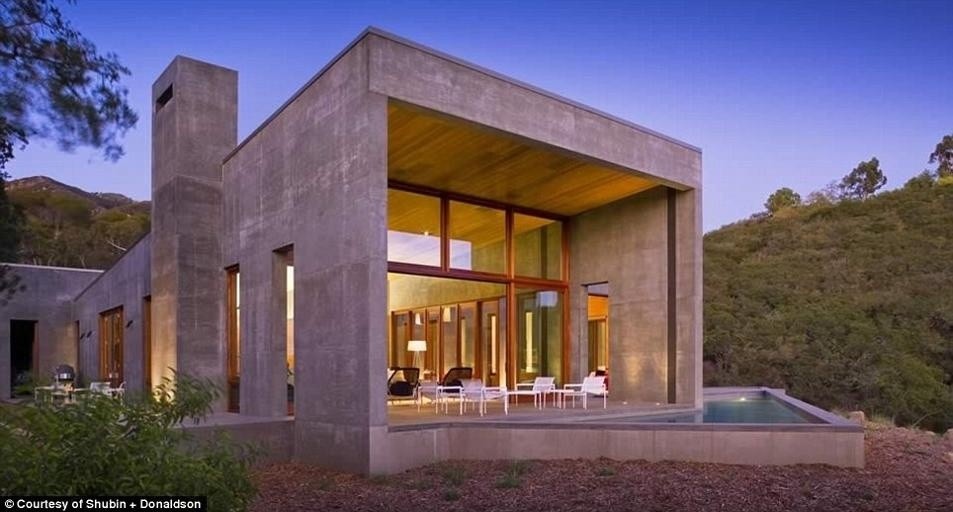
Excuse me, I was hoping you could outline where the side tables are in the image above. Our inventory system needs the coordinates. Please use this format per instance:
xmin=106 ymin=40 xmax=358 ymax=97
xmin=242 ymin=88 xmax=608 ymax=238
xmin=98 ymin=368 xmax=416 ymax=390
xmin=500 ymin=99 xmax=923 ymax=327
xmin=546 ymin=387 xmax=574 ymax=409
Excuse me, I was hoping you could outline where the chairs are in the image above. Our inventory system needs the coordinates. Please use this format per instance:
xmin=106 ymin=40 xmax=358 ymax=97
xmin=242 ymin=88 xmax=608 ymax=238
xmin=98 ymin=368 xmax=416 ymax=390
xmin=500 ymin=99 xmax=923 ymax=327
xmin=388 ymin=367 xmax=423 ymax=409
xmin=34 ymin=381 xmax=126 ymax=405
xmin=563 ymin=376 xmax=608 ymax=410
xmin=507 ymin=376 xmax=556 ymax=409
xmin=418 ymin=368 xmax=508 ymax=416
